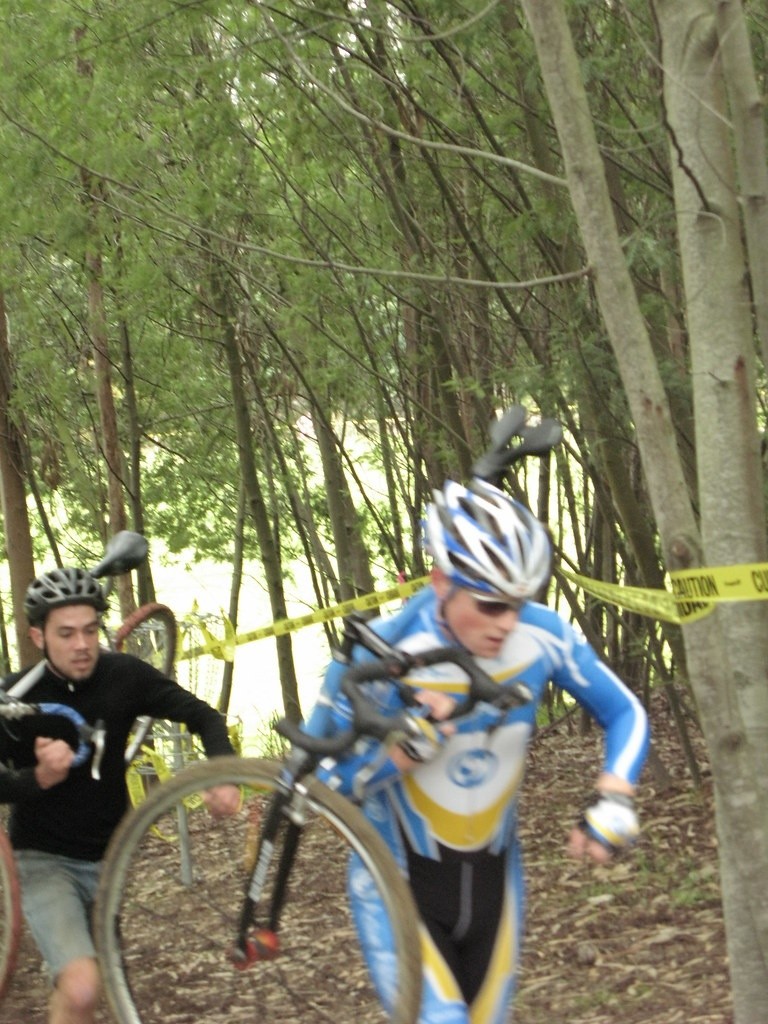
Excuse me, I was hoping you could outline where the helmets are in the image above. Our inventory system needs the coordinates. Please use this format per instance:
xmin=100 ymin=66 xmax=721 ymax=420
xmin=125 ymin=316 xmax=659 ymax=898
xmin=24 ymin=565 xmax=105 ymax=630
xmin=419 ymin=477 xmax=552 ymax=601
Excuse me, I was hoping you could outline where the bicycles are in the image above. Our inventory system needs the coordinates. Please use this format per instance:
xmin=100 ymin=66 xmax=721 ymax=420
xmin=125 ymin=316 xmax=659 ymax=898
xmin=0 ymin=529 xmax=180 ymax=997
xmin=89 ymin=402 xmax=562 ymax=1024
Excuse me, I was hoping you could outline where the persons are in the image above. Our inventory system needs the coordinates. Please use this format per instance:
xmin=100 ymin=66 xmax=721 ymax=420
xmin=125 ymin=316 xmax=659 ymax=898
xmin=0 ymin=566 xmax=244 ymax=1023
xmin=299 ymin=475 xmax=652 ymax=1024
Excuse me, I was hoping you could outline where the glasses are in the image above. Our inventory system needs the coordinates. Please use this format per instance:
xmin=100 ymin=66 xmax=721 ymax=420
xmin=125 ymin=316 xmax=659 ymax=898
xmin=457 ymin=587 xmax=522 ymax=616
